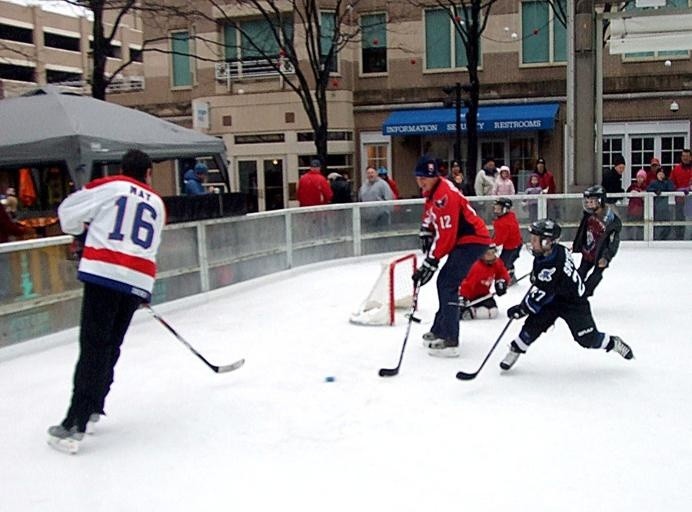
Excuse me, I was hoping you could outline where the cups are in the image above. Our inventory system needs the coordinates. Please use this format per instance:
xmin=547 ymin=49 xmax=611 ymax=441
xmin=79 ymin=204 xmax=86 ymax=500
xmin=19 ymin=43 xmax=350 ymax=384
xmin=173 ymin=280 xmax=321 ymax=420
xmin=213 ymin=188 xmax=220 ymax=193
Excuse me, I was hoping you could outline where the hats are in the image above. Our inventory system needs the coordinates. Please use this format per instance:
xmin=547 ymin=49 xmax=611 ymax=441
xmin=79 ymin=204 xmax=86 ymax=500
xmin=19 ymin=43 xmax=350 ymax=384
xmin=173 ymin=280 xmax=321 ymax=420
xmin=377 ymin=168 xmax=387 ymax=174
xmin=6 ymin=187 xmax=15 ymax=196
xmin=414 ymin=156 xmax=437 ymax=178
xmin=535 ymin=157 xmax=547 ymax=165
xmin=650 ymin=158 xmax=661 ymax=166
xmin=636 ymin=170 xmax=648 ymax=179
xmin=327 ymin=172 xmax=344 ymax=178
xmin=310 ymin=159 xmax=321 ymax=168
xmin=655 ymin=167 xmax=665 ymax=174
xmin=614 ymin=153 xmax=626 ymax=166
xmin=194 ymin=163 xmax=209 ymax=175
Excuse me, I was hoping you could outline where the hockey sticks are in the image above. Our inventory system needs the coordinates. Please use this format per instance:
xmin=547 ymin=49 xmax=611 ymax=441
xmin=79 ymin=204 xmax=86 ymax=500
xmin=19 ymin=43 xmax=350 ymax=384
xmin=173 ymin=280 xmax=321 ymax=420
xmin=145 ymin=302 xmax=244 ymax=374
xmin=456 ymin=282 xmax=535 ymax=378
xmin=378 ymin=281 xmax=420 ymax=378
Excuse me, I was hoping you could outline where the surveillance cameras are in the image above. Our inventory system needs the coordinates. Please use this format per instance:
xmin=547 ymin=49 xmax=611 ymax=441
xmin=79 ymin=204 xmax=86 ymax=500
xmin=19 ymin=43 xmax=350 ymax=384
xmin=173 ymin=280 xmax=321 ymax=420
xmin=670 ymin=101 xmax=679 ymax=112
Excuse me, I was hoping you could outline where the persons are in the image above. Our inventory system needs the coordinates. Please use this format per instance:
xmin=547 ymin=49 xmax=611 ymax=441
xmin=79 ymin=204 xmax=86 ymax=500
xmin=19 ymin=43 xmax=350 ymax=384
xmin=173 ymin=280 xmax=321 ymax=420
xmin=412 ymin=157 xmax=490 ymax=358
xmin=573 ymin=185 xmax=623 ymax=299
xmin=1 ymin=196 xmax=31 ymax=243
xmin=184 ymin=163 xmax=214 ymax=197
xmin=46 ymin=149 xmax=167 ymax=454
xmin=499 ymin=220 xmax=634 ymax=374
xmin=457 ymin=238 xmax=511 ymax=319
xmin=598 ymin=149 xmax=691 ymax=240
xmin=439 ymin=157 xmax=560 ymax=226
xmin=296 ymin=160 xmax=400 ymax=234
xmin=0 ymin=187 xmax=18 ymax=199
xmin=491 ymin=197 xmax=523 ymax=286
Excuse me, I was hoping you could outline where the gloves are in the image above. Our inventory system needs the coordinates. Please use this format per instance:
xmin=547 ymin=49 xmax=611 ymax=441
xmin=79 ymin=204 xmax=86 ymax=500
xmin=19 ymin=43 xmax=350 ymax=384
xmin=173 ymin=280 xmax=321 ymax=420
xmin=506 ymin=304 xmax=527 ymax=321
xmin=412 ymin=257 xmax=440 ymax=288
xmin=417 ymin=227 xmax=435 ymax=253
xmin=493 ymin=278 xmax=508 ymax=296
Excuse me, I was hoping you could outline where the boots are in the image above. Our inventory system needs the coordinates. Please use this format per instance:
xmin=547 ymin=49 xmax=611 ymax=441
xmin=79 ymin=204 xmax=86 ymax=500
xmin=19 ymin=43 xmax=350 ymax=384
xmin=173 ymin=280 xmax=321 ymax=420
xmin=422 ymin=328 xmax=445 ymax=344
xmin=500 ymin=347 xmax=521 ymax=373
xmin=606 ymin=336 xmax=637 ymax=361
xmin=85 ymin=413 xmax=101 ymax=435
xmin=425 ymin=335 xmax=463 ymax=359
xmin=45 ymin=422 xmax=84 ymax=457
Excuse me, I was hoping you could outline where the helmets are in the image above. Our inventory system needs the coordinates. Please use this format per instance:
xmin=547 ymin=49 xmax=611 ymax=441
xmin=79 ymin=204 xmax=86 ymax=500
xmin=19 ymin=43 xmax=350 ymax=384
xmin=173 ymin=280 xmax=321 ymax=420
xmin=525 ymin=220 xmax=562 ymax=257
xmin=492 ymin=198 xmax=513 ymax=218
xmin=582 ymin=185 xmax=607 ymax=214
